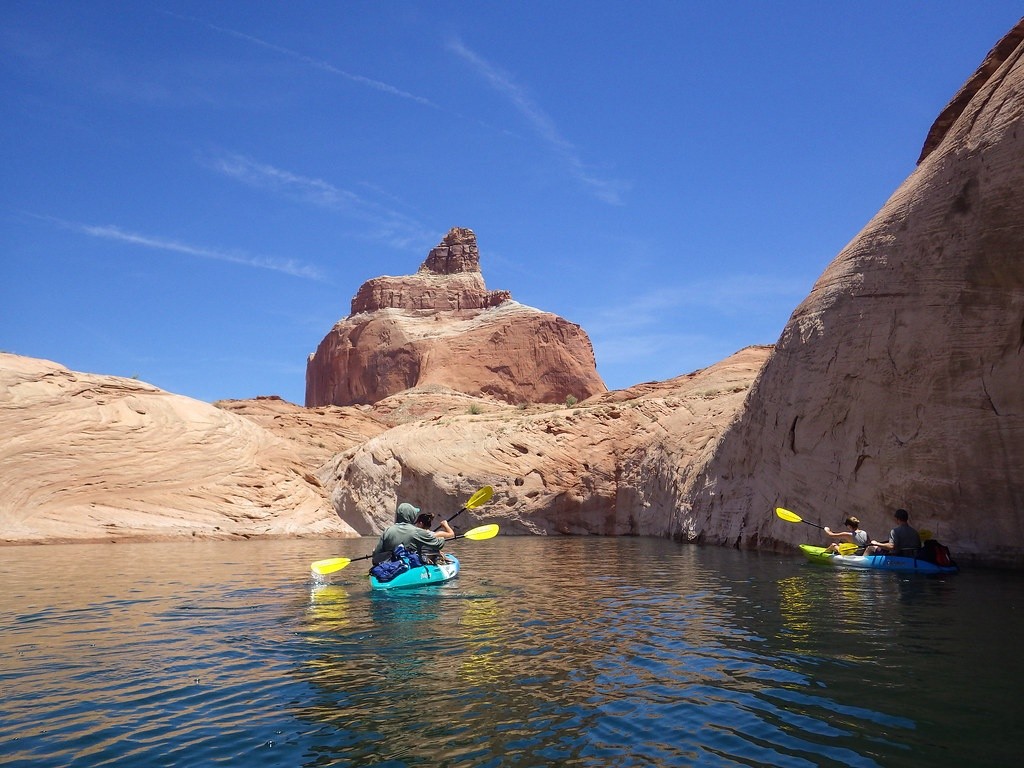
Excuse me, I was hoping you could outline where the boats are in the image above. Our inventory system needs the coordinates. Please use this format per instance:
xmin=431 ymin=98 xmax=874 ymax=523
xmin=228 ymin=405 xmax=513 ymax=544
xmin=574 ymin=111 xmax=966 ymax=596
xmin=368 ymin=554 xmax=461 ymax=590
xmin=799 ymin=543 xmax=958 ymax=575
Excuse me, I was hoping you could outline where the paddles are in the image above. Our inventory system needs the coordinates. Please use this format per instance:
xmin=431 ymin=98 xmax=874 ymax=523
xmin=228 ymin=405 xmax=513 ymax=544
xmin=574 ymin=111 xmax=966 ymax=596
xmin=430 ymin=485 xmax=495 ymax=531
xmin=774 ymin=508 xmax=837 ymax=532
xmin=310 ymin=524 xmax=500 ymax=575
xmin=838 ymin=540 xmax=889 ymax=556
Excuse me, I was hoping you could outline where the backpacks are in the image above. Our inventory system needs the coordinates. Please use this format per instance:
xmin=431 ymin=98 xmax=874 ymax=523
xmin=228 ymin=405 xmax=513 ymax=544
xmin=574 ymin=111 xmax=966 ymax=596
xmin=922 ymin=539 xmax=960 ymax=571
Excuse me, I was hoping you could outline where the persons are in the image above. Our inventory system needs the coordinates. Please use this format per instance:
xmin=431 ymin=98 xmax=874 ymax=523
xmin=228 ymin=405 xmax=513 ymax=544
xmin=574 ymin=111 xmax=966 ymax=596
xmin=372 ymin=502 xmax=456 ymax=564
xmin=823 ymin=509 xmax=922 ymax=558
